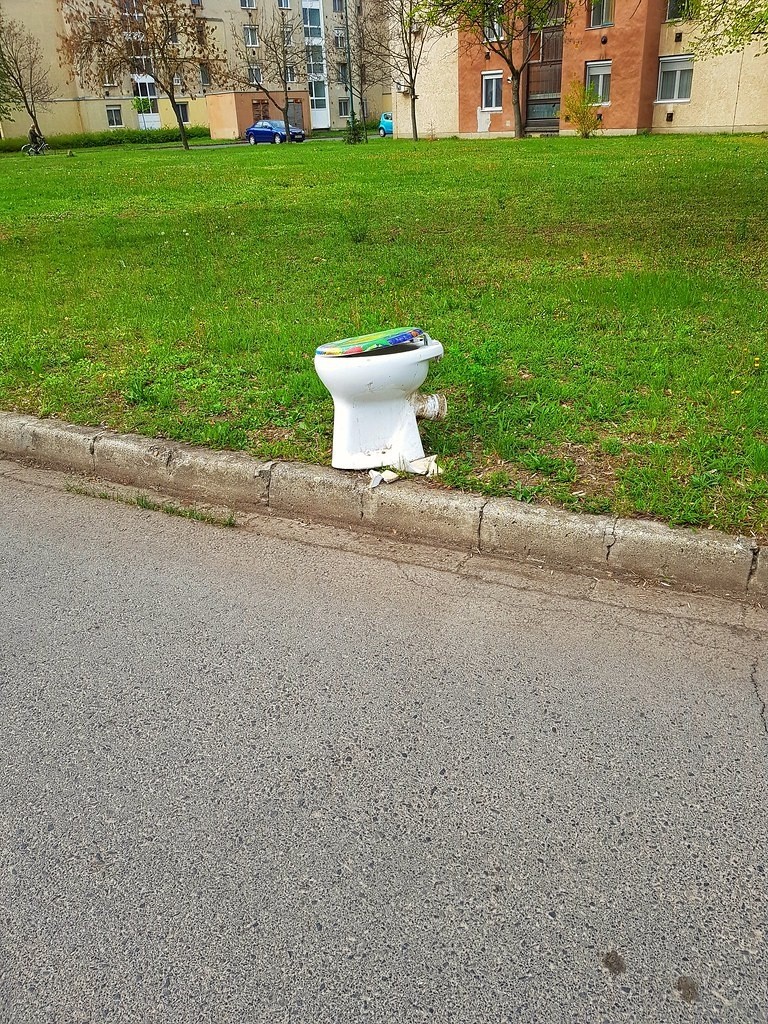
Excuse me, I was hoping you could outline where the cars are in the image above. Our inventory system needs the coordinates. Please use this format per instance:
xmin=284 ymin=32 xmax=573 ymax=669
xmin=378 ymin=112 xmax=393 ymax=137
xmin=246 ymin=120 xmax=306 ymax=146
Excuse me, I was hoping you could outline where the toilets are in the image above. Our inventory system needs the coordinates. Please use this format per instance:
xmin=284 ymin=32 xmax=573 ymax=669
xmin=311 ymin=325 xmax=451 ymax=471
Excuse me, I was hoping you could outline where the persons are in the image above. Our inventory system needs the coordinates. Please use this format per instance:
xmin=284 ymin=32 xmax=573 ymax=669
xmin=27 ymin=124 xmax=45 ymax=154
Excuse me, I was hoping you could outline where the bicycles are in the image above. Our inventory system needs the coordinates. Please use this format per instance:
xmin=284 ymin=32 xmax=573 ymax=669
xmin=21 ymin=136 xmax=53 ymax=156
